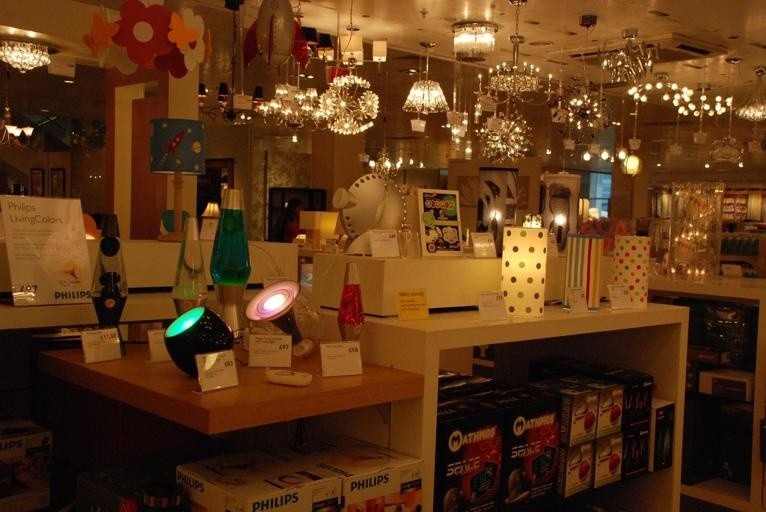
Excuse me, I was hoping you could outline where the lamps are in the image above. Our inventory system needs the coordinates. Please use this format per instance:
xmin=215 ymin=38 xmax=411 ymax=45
xmin=614 ymin=234 xmax=653 ymax=309
xmin=410 ymin=68 xmax=427 ymax=133
xmin=474 ymin=166 xmax=518 ymax=258
xmin=318 ymin=64 xmax=379 ymax=138
xmin=318 ymin=20 xmax=390 ymax=66
xmin=564 ymin=234 xmax=602 ymax=310
xmin=4 ymin=118 xmax=35 ymax=141
xmin=199 ymin=11 xmax=265 ymax=127
xmin=0 ymin=19 xmax=66 ymax=75
xmin=447 ymin=1 xmax=766 ymax=176
xmin=254 ymin=59 xmax=322 ymax=132
xmin=539 ymin=170 xmax=582 ymax=255
xmin=500 ymin=223 xmax=549 ymax=317
xmin=402 ymin=48 xmax=449 ymax=113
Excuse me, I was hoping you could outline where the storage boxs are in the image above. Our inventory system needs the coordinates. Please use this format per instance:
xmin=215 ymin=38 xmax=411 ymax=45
xmin=175 ymin=450 xmax=342 ymax=512
xmin=268 ymin=431 xmax=424 ymax=512
xmin=0 ymin=414 xmax=51 ymax=511
xmin=71 ymin=461 xmax=164 ymax=511
xmin=435 ymin=363 xmax=677 ymax=512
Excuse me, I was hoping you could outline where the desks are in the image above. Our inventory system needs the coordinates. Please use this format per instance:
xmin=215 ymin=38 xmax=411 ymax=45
xmin=34 ymin=344 xmax=424 ymax=512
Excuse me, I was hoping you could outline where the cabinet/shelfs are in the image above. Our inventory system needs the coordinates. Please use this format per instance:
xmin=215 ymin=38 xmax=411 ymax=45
xmin=311 ymin=299 xmax=692 ymax=512
xmin=643 ymin=270 xmax=766 ymax=512
xmin=81 ymin=186 xmax=372 ymax=379
